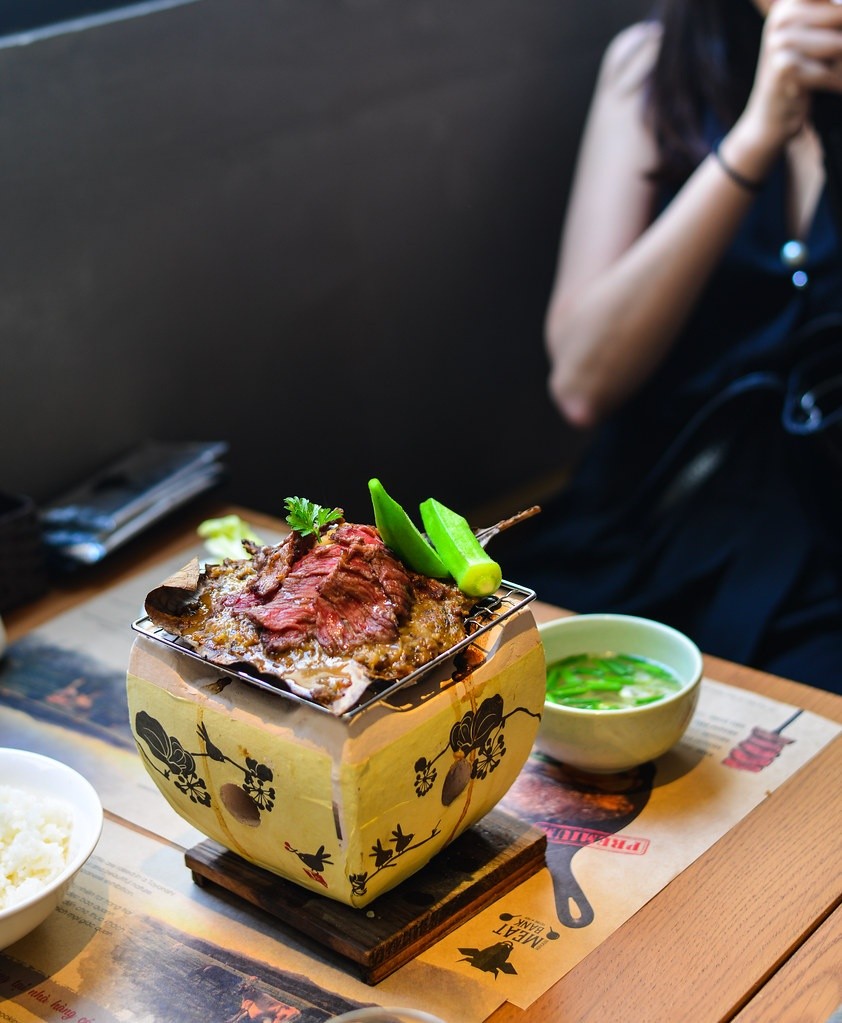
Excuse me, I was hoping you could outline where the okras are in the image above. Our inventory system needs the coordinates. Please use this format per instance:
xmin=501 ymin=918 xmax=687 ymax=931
xmin=365 ymin=477 xmax=501 ymax=596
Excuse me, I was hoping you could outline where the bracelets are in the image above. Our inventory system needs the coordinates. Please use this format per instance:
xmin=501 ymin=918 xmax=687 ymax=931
xmin=713 ymin=138 xmax=759 ymax=192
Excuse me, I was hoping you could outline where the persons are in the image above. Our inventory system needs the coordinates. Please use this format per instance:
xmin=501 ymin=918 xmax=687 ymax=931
xmin=542 ymin=0 xmax=842 ymax=698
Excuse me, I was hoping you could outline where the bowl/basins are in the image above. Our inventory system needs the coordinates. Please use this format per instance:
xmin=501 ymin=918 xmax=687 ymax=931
xmin=0 ymin=746 xmax=103 ymax=954
xmin=526 ymin=610 xmax=710 ymax=779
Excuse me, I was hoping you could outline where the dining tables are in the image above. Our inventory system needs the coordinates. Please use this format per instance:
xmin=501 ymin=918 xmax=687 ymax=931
xmin=1 ymin=495 xmax=842 ymax=1022
